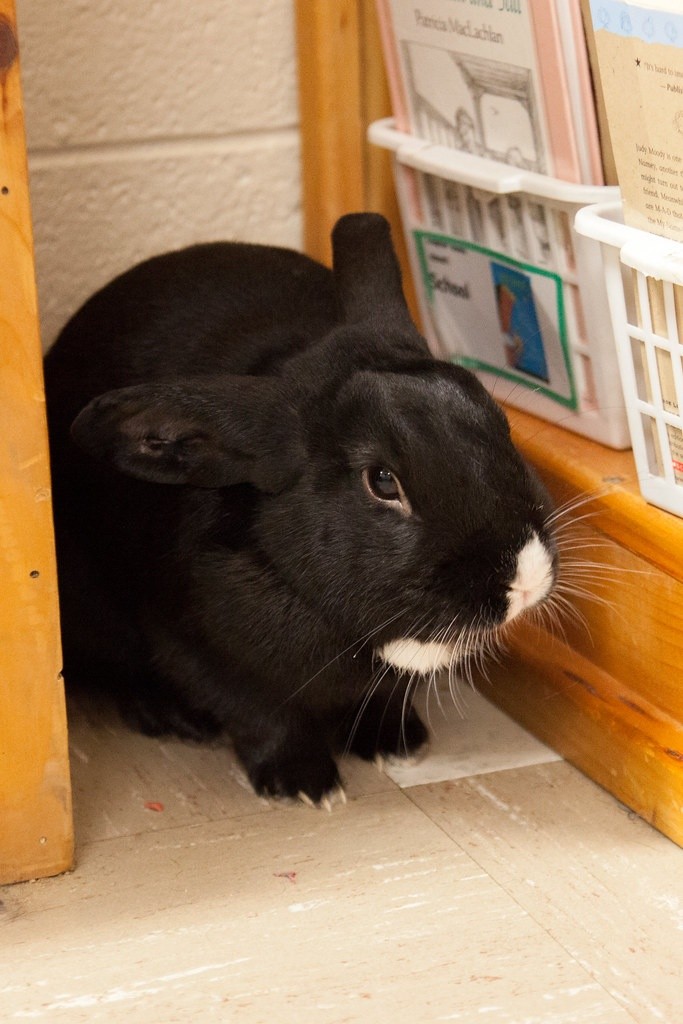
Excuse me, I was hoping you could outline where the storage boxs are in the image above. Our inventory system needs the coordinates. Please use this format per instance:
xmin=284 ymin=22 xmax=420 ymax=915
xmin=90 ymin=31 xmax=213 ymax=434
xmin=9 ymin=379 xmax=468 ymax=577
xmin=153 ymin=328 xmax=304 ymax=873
xmin=572 ymin=195 xmax=683 ymax=520
xmin=365 ymin=110 xmax=634 ymax=455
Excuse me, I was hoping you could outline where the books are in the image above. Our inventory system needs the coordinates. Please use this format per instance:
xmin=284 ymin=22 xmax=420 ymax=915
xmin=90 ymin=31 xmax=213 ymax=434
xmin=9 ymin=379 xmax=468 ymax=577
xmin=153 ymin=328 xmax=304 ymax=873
xmin=377 ymin=0 xmax=683 ymax=487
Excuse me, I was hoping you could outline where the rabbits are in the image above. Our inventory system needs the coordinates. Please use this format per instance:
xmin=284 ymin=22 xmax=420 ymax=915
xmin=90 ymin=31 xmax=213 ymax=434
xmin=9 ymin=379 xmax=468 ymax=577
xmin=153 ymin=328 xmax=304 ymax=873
xmin=40 ymin=212 xmax=658 ymax=814
